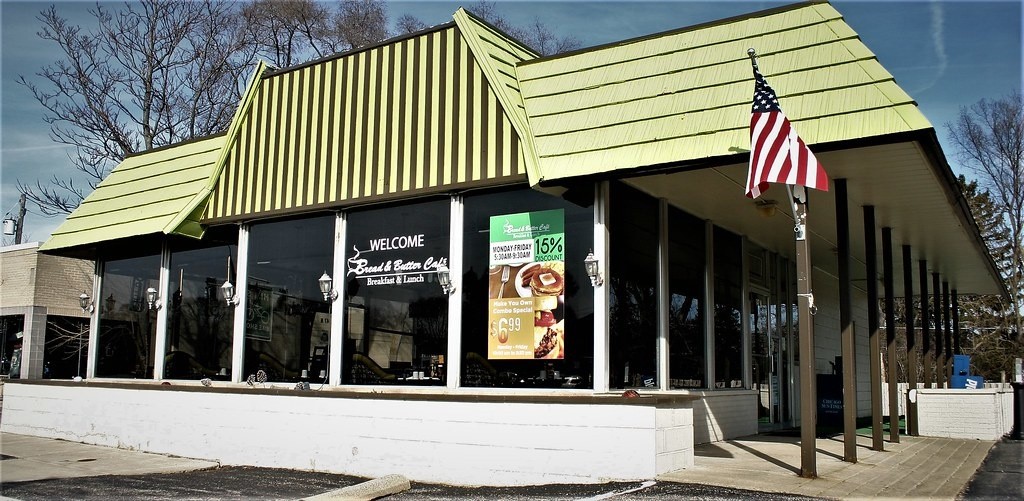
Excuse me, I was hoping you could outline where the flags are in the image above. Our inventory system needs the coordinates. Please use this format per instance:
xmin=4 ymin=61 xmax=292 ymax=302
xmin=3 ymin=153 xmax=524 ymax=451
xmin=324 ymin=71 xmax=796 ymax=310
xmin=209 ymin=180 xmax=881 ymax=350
xmin=744 ymin=64 xmax=830 ymax=199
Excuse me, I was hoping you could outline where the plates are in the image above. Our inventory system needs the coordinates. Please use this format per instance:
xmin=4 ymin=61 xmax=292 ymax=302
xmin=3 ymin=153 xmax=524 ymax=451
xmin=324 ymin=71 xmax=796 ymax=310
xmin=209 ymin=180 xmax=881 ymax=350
xmin=515 ymin=261 xmax=565 ymax=298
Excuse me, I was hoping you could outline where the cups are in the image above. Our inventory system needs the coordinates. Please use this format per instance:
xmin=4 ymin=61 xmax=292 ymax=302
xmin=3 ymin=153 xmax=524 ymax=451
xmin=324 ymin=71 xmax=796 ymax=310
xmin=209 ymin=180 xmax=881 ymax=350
xmin=302 ymin=370 xmax=307 ymax=377
xmin=221 ymin=368 xmax=225 ymax=375
xmin=413 ymin=372 xmax=418 ymax=379
xmin=419 ymin=372 xmax=424 ymax=379
xmin=321 ymin=370 xmax=325 ymax=377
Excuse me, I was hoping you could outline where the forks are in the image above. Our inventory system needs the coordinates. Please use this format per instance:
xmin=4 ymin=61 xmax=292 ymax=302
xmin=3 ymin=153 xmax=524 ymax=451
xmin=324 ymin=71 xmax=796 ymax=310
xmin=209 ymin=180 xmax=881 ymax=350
xmin=497 ymin=266 xmax=511 ymax=300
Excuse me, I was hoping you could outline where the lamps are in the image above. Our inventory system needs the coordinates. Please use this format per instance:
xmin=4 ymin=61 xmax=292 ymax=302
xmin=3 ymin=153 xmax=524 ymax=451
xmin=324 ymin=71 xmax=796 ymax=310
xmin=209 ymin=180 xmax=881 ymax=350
xmin=319 ymin=269 xmax=337 ymax=301
xmin=437 ymin=263 xmax=455 ymax=294
xmin=756 ymin=201 xmax=777 ymax=218
xmin=221 ymin=279 xmax=239 ymax=306
xmin=146 ymin=285 xmax=161 ymax=310
xmin=79 ymin=290 xmax=94 ymax=314
